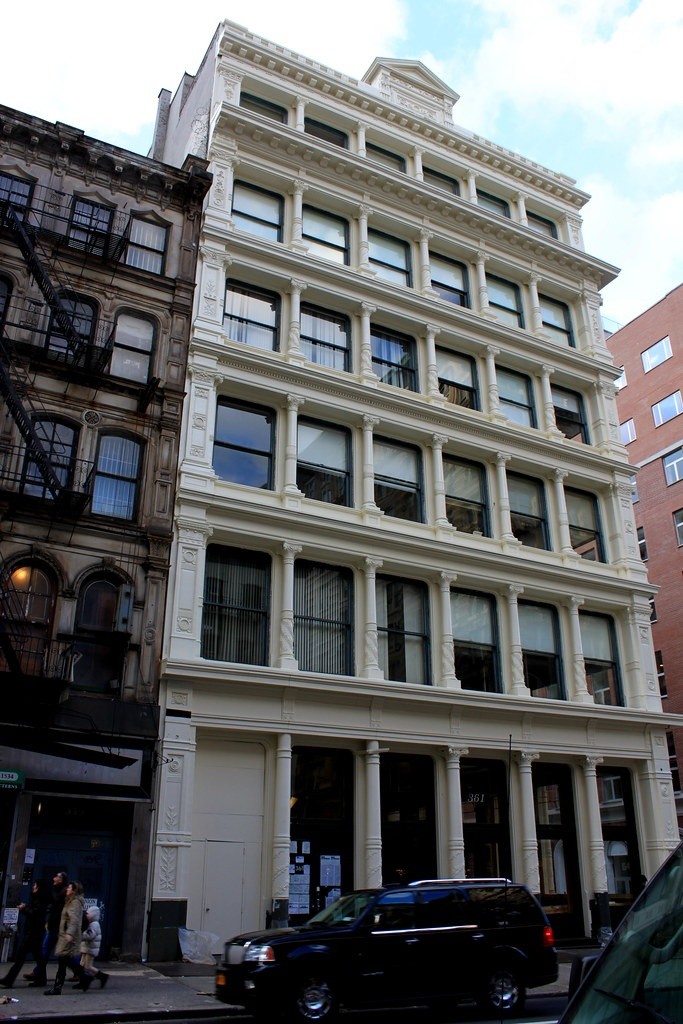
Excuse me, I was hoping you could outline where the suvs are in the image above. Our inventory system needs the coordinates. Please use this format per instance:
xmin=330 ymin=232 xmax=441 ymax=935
xmin=213 ymin=877 xmax=560 ymax=1024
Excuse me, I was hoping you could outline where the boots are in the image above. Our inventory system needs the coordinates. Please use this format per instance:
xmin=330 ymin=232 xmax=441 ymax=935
xmin=72 ymin=969 xmax=89 ymax=989
xmin=72 ymin=979 xmax=84 ymax=989
xmin=95 ymin=970 xmax=109 ymax=988
xmin=43 ymin=971 xmax=66 ymax=996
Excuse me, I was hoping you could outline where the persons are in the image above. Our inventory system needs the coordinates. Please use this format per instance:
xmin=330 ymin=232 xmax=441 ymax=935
xmin=633 ymin=875 xmax=648 ymax=904
xmin=79 ymin=907 xmax=110 ymax=992
xmin=42 ymin=881 xmax=93 ymax=996
xmin=1 ymin=879 xmax=52 ymax=989
xmin=23 ymin=870 xmax=86 ymax=983
